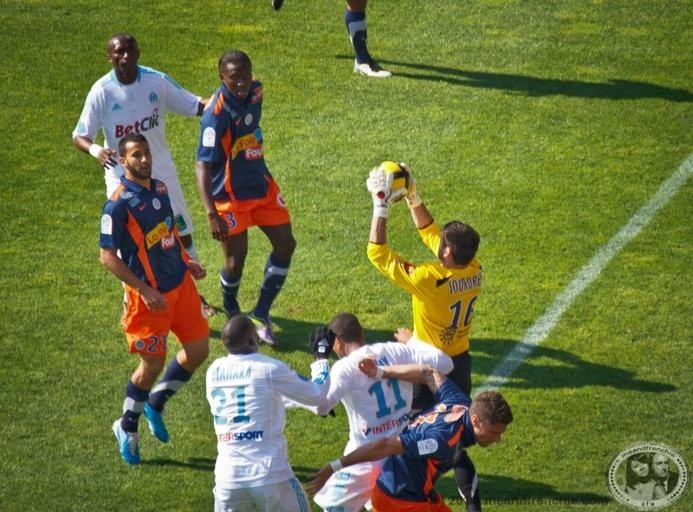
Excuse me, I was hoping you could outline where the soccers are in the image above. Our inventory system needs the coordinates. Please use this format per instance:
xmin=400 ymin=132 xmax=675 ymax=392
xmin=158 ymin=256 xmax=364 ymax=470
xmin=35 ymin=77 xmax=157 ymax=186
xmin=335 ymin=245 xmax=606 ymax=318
xmin=373 ymin=160 xmax=410 ymax=202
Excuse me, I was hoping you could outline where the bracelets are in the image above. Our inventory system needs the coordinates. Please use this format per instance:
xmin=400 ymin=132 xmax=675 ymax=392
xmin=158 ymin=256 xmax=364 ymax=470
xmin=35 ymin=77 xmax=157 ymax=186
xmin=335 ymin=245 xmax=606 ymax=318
xmin=87 ymin=144 xmax=103 ymax=157
xmin=328 ymin=458 xmax=344 ymax=471
xmin=374 ymin=366 xmax=383 ymax=378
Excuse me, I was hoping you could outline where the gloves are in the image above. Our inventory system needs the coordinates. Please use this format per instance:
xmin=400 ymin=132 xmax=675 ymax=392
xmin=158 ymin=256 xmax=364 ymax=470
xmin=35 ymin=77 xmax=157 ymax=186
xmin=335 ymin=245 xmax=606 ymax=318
xmin=400 ymin=161 xmax=422 ymax=209
xmin=366 ymin=167 xmax=404 ymax=219
xmin=309 ymin=325 xmax=337 ymax=358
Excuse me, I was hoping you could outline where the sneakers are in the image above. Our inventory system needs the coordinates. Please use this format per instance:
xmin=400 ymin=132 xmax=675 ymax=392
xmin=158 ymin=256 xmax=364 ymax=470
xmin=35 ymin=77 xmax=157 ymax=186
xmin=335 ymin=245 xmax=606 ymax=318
xmin=112 ymin=417 xmax=140 ymax=465
xmin=354 ymin=57 xmax=391 ymax=78
xmin=248 ymin=311 xmax=277 ymax=347
xmin=199 ymin=295 xmax=215 ymax=317
xmin=143 ymin=403 xmax=169 ymax=442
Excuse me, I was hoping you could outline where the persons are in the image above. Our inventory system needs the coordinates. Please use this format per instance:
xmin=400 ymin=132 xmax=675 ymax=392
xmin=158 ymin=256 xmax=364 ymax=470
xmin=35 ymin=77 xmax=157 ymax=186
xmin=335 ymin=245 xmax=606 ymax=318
xmin=277 ymin=314 xmax=455 ymax=512
xmin=99 ymin=132 xmax=210 ymax=464
xmin=366 ymin=161 xmax=483 ymax=511
xmin=71 ymin=31 xmax=208 ymax=287
xmin=196 ymin=49 xmax=294 ymax=348
xmin=201 ymin=313 xmax=333 ymax=511
xmin=271 ymin=0 xmax=392 ymax=76
xmin=625 ymin=453 xmax=660 ymax=500
xmin=305 ymin=356 xmax=513 ymax=512
xmin=648 ymin=453 xmax=677 ymax=495
